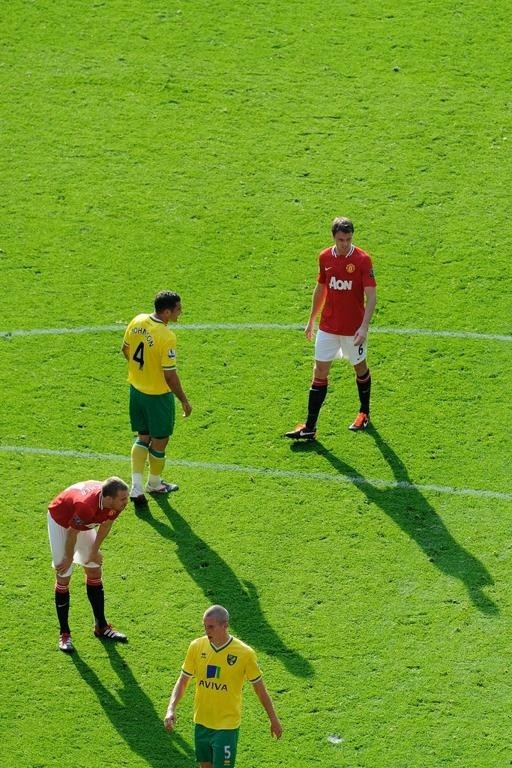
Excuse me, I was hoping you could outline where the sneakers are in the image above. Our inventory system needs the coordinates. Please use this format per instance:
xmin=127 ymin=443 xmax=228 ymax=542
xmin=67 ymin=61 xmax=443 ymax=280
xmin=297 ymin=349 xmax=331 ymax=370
xmin=57 ymin=630 xmax=75 ymax=653
xmin=348 ymin=411 xmax=370 ymax=430
xmin=93 ymin=625 xmax=127 ymax=642
xmin=145 ymin=481 xmax=179 ymax=493
xmin=284 ymin=426 xmax=318 ymax=440
xmin=129 ymin=486 xmax=145 ymax=500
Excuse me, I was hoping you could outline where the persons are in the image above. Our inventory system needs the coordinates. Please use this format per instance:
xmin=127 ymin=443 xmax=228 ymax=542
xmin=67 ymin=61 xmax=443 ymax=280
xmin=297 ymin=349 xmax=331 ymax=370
xmin=161 ymin=602 xmax=283 ymax=768
xmin=281 ymin=214 xmax=379 ymax=441
xmin=120 ymin=291 xmax=192 ymax=500
xmin=44 ymin=475 xmax=132 ymax=654
